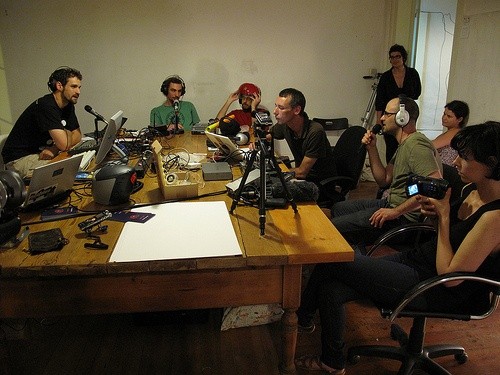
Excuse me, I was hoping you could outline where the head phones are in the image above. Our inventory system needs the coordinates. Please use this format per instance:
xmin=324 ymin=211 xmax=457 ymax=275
xmin=47 ymin=66 xmax=70 ymax=92
xmin=229 ymin=132 xmax=251 ymax=146
xmin=395 ymin=94 xmax=410 ymax=128
xmin=160 ymin=74 xmax=186 ymax=96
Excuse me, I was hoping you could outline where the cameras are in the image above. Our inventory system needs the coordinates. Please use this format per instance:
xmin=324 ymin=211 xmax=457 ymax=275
xmin=404 ymin=175 xmax=451 ymax=200
xmin=255 ymin=112 xmax=273 ymax=126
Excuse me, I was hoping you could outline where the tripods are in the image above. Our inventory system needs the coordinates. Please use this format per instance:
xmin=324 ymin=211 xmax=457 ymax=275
xmin=230 ymin=121 xmax=298 ymax=235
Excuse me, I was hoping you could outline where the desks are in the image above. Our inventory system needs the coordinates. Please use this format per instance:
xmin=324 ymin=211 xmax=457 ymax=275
xmin=0 ymin=129 xmax=355 ymax=375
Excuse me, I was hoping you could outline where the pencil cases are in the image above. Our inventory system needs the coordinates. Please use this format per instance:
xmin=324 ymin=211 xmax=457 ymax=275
xmin=29 ymin=228 xmax=68 ymax=255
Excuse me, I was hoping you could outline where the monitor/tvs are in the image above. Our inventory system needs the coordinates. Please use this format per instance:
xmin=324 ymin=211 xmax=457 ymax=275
xmin=95 ymin=111 xmax=129 ymax=165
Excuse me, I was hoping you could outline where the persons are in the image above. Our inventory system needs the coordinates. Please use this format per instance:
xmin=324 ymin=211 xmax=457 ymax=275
xmin=248 ymin=88 xmax=354 ymax=208
xmin=215 ymin=84 xmax=266 ymax=127
xmin=149 ymin=77 xmax=200 ymax=132
xmin=375 ymin=44 xmax=422 ymax=165
xmin=2 ymin=69 xmax=82 ymax=165
xmin=327 ymin=97 xmax=443 ymax=303
xmin=272 ymin=120 xmax=500 ymax=375
xmin=380 ymin=101 xmax=469 ymax=198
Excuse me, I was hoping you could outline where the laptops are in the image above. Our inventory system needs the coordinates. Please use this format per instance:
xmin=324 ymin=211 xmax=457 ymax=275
xmin=16 ymin=154 xmax=84 ymax=213
xmin=67 ymin=117 xmax=127 ymax=157
xmin=205 ymin=132 xmax=261 ymax=161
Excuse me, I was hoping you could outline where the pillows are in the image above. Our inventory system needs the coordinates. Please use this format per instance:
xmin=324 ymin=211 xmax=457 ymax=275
xmin=221 ymin=304 xmax=285 ymax=332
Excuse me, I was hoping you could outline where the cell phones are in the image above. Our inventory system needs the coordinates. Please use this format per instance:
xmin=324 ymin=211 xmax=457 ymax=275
xmin=78 ymin=212 xmax=109 ymax=231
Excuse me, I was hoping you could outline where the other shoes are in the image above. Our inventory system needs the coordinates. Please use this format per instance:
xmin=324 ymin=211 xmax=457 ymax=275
xmin=295 ymin=354 xmax=345 ymax=375
xmin=297 ymin=322 xmax=316 ymax=334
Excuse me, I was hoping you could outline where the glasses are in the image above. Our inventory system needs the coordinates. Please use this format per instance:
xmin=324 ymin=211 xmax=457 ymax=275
xmin=388 ymin=55 xmax=402 ymax=59
xmin=383 ymin=110 xmax=394 ymax=116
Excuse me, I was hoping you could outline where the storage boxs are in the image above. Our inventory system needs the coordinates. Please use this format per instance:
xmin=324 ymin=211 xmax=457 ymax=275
xmin=151 ymin=140 xmax=199 ymax=201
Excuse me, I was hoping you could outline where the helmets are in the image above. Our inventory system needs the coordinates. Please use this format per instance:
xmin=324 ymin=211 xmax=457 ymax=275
xmin=239 ymin=83 xmax=259 ymax=95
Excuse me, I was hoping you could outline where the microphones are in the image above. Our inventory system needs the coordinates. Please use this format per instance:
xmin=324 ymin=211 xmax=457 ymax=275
xmin=173 ymin=99 xmax=179 ymax=111
xmin=84 ymin=105 xmax=108 ymax=125
xmin=359 ymin=124 xmax=382 ymax=152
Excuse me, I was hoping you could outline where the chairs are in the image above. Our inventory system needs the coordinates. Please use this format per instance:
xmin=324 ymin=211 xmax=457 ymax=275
xmin=374 ymin=162 xmax=466 ymax=254
xmin=348 ymin=182 xmax=500 ymax=375
xmin=285 ymin=124 xmax=368 ymax=218
xmin=313 ymin=118 xmax=348 ymax=148
